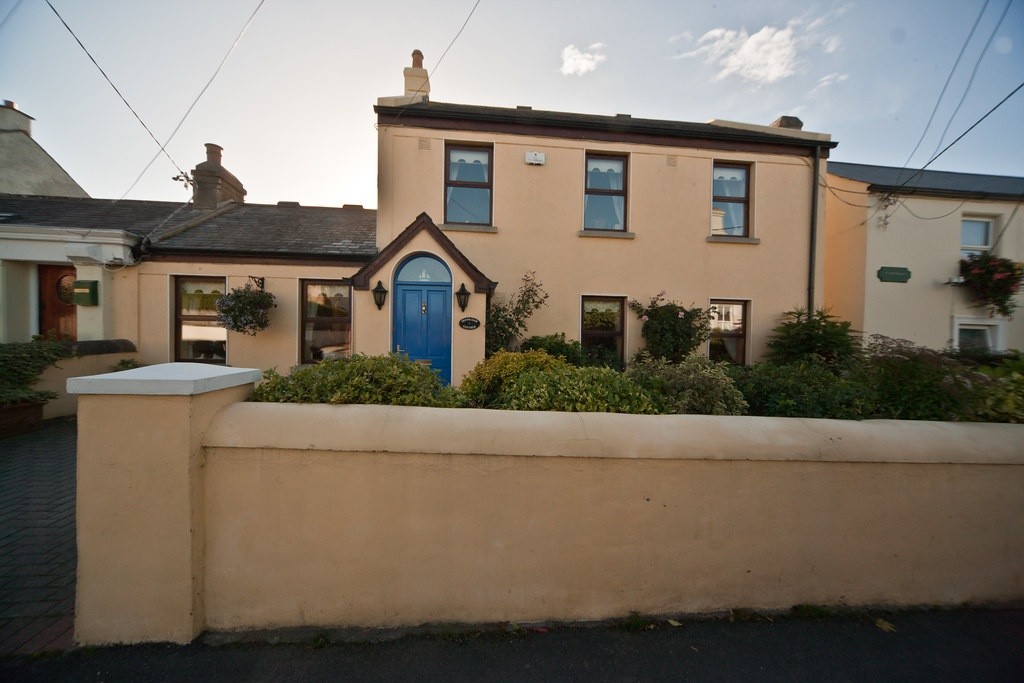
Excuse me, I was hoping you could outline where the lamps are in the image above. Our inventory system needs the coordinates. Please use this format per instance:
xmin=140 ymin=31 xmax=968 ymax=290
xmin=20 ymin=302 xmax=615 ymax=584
xmin=454 ymin=283 xmax=471 ymax=312
xmin=371 ymin=281 xmax=388 ymax=310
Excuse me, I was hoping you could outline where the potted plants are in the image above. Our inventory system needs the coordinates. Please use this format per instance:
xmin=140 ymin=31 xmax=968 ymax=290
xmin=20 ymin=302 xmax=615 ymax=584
xmin=0 ymin=331 xmax=75 ymax=438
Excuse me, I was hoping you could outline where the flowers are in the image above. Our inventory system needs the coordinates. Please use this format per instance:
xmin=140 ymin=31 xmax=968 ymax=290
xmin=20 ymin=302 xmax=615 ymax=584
xmin=957 ymin=252 xmax=1024 ymax=321
